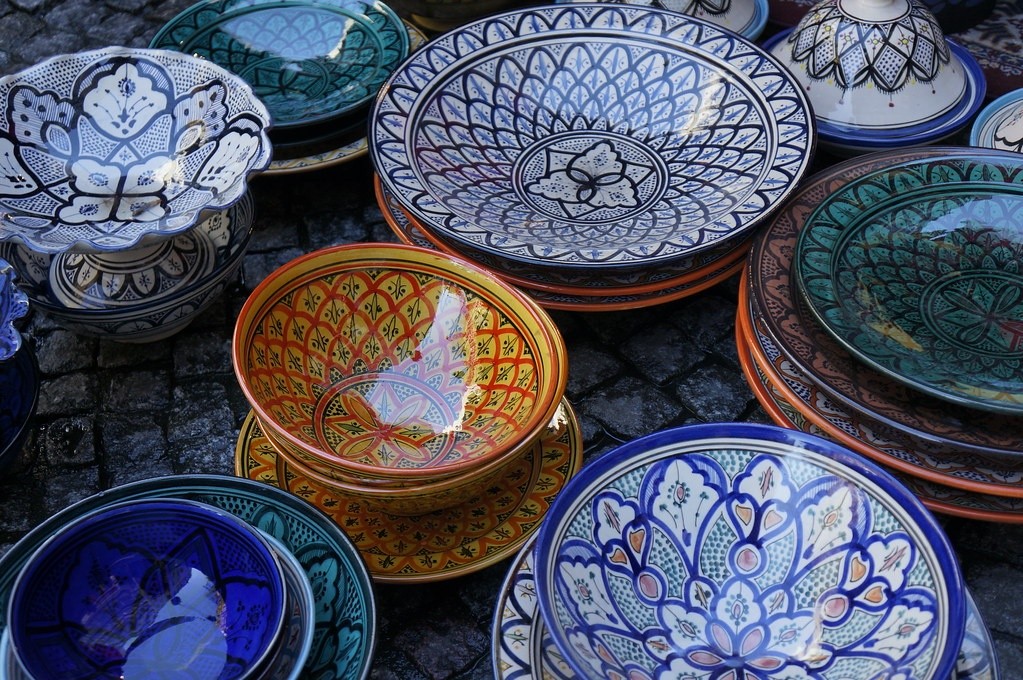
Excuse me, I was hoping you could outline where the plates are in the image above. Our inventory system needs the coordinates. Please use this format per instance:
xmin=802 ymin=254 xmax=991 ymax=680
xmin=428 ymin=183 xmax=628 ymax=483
xmin=736 ymin=152 xmax=1023 ymax=523
xmin=487 ymin=526 xmax=1000 ymax=680
xmin=236 ymin=394 xmax=582 ymax=583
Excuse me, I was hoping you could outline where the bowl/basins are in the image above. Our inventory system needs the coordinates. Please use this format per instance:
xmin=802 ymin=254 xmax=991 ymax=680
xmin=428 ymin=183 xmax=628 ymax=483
xmin=372 ymin=0 xmax=818 ymax=312
xmin=0 ymin=475 xmax=377 ymax=680
xmin=228 ymin=243 xmax=568 ymax=520
xmin=757 ymin=0 xmax=1022 ymax=153
xmin=534 ymin=420 xmax=967 ymax=680
xmin=796 ymin=156 xmax=1022 ymax=415
xmin=1 ymin=0 xmax=434 ymax=467
